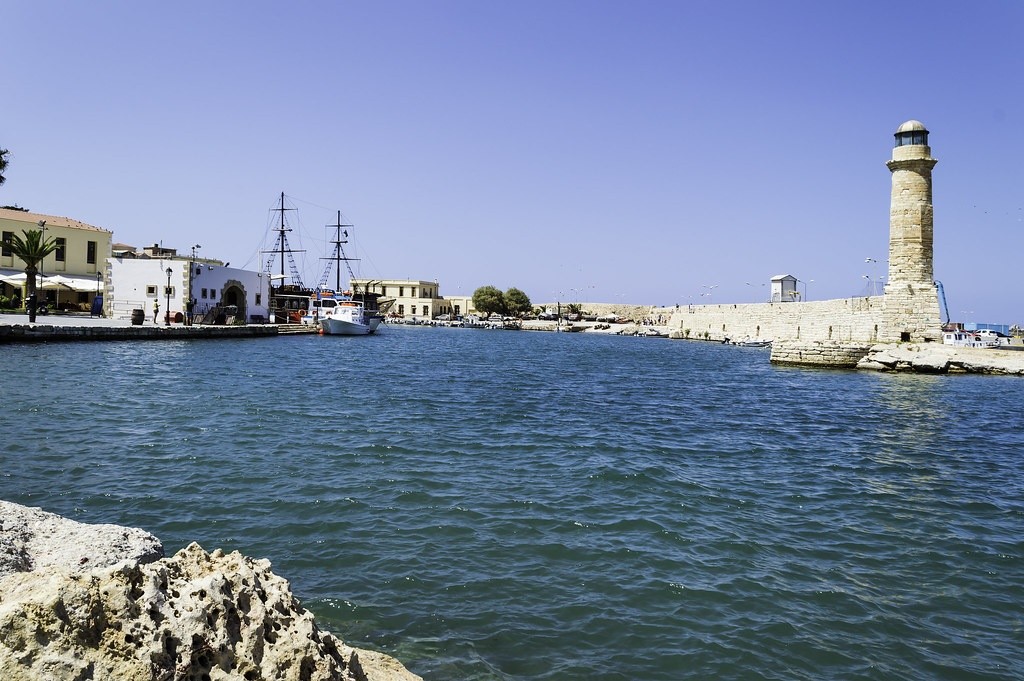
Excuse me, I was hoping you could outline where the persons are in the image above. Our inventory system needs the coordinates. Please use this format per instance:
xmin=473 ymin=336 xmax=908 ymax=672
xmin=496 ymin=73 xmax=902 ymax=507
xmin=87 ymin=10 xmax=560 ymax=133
xmin=187 ymin=300 xmax=194 ymax=326
xmin=642 ymin=314 xmax=662 ymax=326
xmin=153 ymin=299 xmax=160 ymax=324
xmin=508 ymin=317 xmax=522 ymax=328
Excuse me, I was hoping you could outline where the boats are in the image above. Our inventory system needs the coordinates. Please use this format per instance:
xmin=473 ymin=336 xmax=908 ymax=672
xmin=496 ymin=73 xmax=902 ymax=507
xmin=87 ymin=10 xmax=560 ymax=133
xmin=380 ymin=318 xmax=519 ymax=329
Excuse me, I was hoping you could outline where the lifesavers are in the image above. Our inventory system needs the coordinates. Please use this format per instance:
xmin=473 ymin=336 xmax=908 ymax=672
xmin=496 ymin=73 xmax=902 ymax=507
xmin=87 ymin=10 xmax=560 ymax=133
xmin=299 ymin=309 xmax=306 ymax=317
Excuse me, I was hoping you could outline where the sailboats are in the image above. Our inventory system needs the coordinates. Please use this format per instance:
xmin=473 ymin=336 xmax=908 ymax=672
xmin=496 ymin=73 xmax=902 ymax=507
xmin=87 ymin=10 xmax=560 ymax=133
xmin=238 ymin=189 xmax=396 ymax=335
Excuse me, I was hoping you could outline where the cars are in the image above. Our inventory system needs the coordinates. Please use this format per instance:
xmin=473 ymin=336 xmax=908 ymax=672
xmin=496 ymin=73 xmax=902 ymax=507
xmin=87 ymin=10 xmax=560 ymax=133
xmin=434 ymin=309 xmax=634 ymax=325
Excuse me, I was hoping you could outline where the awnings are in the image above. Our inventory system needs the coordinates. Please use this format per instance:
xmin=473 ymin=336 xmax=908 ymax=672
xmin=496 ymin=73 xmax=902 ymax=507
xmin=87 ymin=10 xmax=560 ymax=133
xmin=0 ymin=269 xmax=105 ymax=308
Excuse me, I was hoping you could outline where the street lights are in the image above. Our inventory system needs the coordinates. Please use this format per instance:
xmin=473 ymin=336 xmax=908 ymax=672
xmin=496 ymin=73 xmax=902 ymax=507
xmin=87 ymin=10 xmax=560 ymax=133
xmin=166 ymin=266 xmax=173 ymax=326
xmin=558 ymin=285 xmax=596 ymax=303
xmin=679 ymin=279 xmax=821 ymax=305
xmin=38 ymin=220 xmax=47 ymax=288
xmin=96 ymin=270 xmax=102 ymax=297
xmin=862 ymin=257 xmax=888 ymax=299
